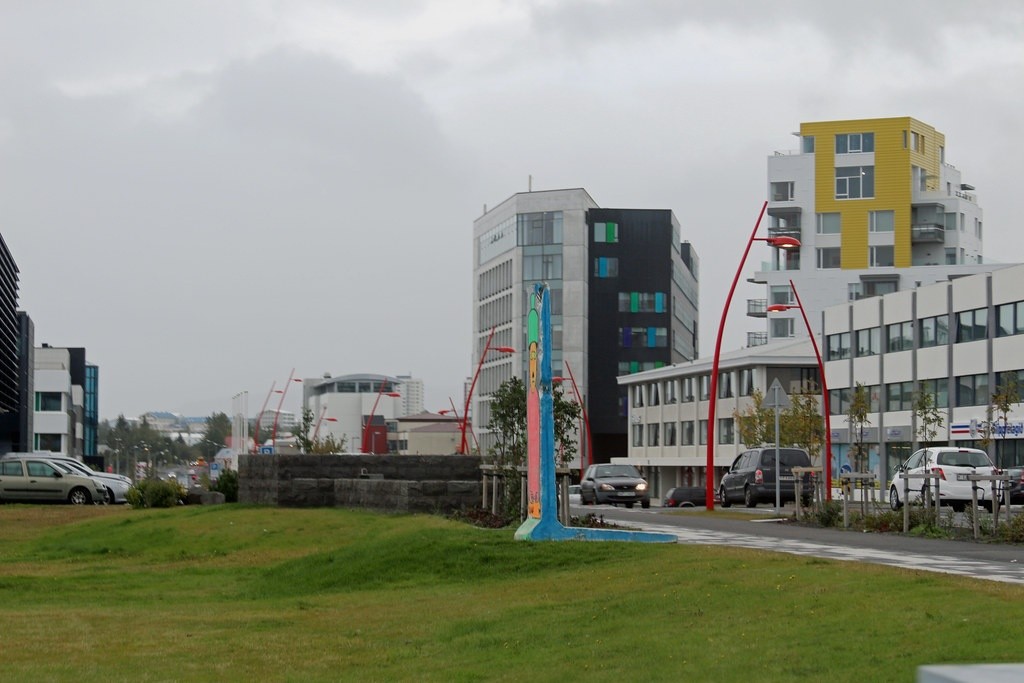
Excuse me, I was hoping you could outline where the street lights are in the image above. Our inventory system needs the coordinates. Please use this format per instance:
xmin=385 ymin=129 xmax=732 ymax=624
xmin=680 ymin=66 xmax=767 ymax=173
xmin=768 ymin=279 xmax=830 ymax=501
xmin=552 ymin=360 xmax=593 ymax=478
xmin=115 ymin=438 xmax=122 ymax=474
xmin=311 ymin=405 xmax=338 ymax=443
xmin=706 ymin=200 xmax=800 ymax=512
xmin=363 ymin=377 xmax=401 ymax=452
xmin=254 ymin=368 xmax=302 ymax=454
xmin=438 ymin=324 xmax=517 ymax=456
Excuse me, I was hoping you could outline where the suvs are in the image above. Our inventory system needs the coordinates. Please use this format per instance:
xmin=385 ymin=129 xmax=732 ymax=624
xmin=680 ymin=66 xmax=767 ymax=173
xmin=717 ymin=447 xmax=816 ymax=507
xmin=663 ymin=487 xmax=720 ymax=507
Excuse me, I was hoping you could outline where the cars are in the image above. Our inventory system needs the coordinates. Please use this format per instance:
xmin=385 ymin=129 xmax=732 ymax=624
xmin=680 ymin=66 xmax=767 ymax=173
xmin=167 ymin=471 xmax=176 ymax=478
xmin=0 ymin=450 xmax=135 ymax=505
xmin=889 ymin=447 xmax=1024 ymax=511
xmin=562 ymin=462 xmax=651 ymax=508
